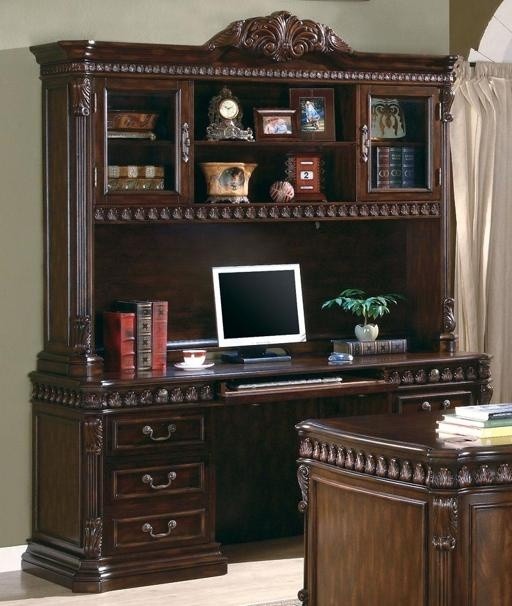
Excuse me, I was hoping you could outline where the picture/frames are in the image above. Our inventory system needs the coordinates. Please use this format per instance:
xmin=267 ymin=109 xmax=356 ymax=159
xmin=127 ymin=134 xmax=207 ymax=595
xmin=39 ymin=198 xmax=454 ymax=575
xmin=250 ymin=87 xmax=336 ymax=140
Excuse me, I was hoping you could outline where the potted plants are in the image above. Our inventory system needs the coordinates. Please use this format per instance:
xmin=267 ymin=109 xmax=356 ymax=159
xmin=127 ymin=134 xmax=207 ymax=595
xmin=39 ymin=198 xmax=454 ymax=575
xmin=320 ymin=286 xmax=409 ymax=342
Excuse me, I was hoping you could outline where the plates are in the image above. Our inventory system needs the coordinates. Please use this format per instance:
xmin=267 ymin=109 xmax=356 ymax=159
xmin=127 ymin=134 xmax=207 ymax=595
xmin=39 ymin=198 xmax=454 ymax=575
xmin=174 ymin=362 xmax=215 ymax=371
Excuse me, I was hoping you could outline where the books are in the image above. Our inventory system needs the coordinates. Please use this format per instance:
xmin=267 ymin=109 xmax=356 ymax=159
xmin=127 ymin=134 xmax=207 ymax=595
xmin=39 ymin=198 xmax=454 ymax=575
xmin=330 ymin=335 xmax=410 ymax=357
xmin=435 ymin=401 xmax=512 ymax=449
xmin=370 ymin=146 xmax=419 ymax=187
xmin=106 ymin=165 xmax=164 ymax=192
xmin=100 ymin=296 xmax=168 ymax=378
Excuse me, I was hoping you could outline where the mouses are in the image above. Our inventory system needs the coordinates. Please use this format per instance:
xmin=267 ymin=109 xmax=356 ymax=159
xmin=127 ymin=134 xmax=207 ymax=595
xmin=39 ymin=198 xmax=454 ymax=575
xmin=328 ymin=352 xmax=353 ymax=362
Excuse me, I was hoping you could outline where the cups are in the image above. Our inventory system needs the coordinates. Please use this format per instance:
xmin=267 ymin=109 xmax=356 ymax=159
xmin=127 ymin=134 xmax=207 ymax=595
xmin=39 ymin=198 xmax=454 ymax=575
xmin=184 ymin=349 xmax=207 ymax=364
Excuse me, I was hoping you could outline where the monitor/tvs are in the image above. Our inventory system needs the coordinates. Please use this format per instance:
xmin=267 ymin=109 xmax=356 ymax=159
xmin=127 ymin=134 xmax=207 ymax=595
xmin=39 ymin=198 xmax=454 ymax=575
xmin=212 ymin=263 xmax=307 ymax=364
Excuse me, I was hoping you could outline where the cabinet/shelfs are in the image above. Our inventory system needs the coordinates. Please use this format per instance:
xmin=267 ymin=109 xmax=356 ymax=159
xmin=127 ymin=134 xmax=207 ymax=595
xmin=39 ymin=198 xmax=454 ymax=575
xmin=21 ymin=9 xmax=492 ymax=593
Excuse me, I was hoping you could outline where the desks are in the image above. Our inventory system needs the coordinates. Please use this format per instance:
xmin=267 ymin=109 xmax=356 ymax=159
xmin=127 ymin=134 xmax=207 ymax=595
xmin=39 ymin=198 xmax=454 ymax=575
xmin=291 ymin=402 xmax=510 ymax=604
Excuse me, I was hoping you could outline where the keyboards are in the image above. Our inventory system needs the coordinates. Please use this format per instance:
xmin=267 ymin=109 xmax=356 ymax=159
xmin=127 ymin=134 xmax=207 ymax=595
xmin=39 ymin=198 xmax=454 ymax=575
xmin=226 ymin=375 xmax=342 ymax=390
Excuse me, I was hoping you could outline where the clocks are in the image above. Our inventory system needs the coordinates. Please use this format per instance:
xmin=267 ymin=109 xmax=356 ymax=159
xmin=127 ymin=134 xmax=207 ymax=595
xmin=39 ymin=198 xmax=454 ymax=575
xmin=206 ymin=83 xmax=253 ymax=142
